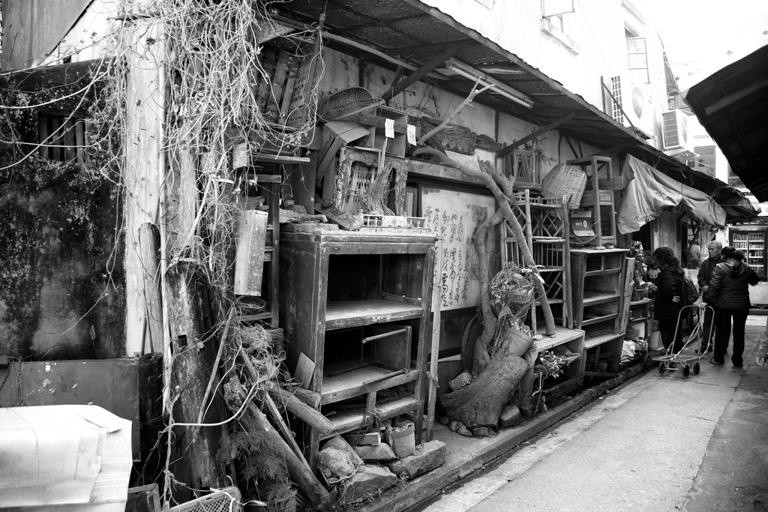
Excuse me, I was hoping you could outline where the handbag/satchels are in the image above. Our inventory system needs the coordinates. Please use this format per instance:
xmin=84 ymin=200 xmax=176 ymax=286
xmin=685 ymin=280 xmax=698 ymax=304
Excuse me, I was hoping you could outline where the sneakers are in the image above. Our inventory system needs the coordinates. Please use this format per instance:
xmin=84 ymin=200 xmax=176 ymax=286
xmin=709 ymin=357 xmax=721 ymax=365
xmin=695 ymin=345 xmax=713 ymax=354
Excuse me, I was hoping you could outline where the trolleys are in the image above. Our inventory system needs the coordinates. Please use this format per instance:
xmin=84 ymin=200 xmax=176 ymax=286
xmin=651 ymin=303 xmax=715 ymax=377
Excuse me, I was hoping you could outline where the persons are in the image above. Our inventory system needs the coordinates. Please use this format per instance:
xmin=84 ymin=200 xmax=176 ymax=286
xmin=645 ymin=245 xmax=693 ymax=372
xmin=702 ymin=245 xmax=760 ymax=369
xmin=696 ymin=239 xmax=729 ymax=355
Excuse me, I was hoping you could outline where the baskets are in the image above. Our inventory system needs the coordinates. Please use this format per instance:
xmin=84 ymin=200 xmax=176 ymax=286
xmin=320 ymin=86 xmax=385 ymax=124
xmin=540 ymin=164 xmax=588 ymax=210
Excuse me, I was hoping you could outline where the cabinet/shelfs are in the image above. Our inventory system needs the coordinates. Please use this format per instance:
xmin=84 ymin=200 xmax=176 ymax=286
xmin=275 ymin=223 xmax=439 ymax=473
xmin=727 ymin=224 xmax=767 ymax=282
xmin=499 ymin=155 xmax=631 ymax=414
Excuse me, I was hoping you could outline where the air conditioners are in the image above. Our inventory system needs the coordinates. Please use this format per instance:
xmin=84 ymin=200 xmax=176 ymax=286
xmin=609 ymin=74 xmax=696 ymax=158
